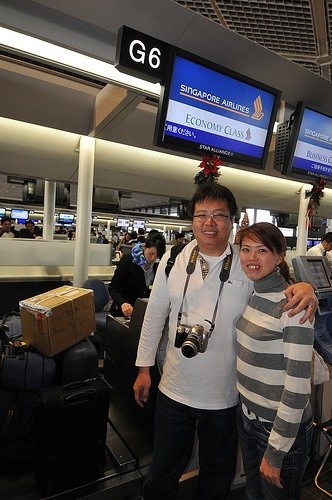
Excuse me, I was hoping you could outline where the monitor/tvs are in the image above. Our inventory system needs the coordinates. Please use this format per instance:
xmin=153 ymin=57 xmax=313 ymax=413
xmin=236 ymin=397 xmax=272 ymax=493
xmin=58 ymin=214 xmax=74 ymax=223
xmin=292 ymin=256 xmax=332 ymax=292
xmin=152 ymin=45 xmax=282 ymax=171
xmin=0 ymin=208 xmax=6 ymax=218
xmin=273 ymin=102 xmax=332 ymax=183
xmin=10 ymin=210 xmax=29 ymax=220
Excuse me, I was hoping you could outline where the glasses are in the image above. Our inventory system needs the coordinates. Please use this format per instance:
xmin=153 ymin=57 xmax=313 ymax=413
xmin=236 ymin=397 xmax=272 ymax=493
xmin=192 ymin=212 xmax=231 ymax=222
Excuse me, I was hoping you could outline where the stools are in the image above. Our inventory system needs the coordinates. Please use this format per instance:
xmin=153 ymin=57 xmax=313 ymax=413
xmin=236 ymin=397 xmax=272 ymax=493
xmin=312 ymin=417 xmax=332 ymax=498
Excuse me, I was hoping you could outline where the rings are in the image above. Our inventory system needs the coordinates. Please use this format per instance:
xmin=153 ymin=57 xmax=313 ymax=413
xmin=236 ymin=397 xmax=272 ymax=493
xmin=308 ymin=304 xmax=314 ymax=309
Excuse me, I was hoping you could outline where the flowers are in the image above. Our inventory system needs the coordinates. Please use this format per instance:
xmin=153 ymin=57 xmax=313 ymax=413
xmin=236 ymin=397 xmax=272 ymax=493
xmin=303 ymin=174 xmax=328 ymax=213
xmin=193 ymin=154 xmax=223 ymax=187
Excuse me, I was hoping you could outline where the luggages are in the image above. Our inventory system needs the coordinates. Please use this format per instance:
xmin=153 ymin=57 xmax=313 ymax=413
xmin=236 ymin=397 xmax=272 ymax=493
xmin=0 ymin=345 xmax=56 ymax=390
xmin=0 ymin=387 xmax=45 ymax=482
xmin=34 ymin=374 xmax=110 ymax=500
xmin=65 ymin=338 xmax=102 ymax=374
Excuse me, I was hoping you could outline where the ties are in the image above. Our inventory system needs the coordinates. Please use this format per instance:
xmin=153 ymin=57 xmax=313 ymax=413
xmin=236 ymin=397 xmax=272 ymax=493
xmin=323 ymin=251 xmax=326 ymax=256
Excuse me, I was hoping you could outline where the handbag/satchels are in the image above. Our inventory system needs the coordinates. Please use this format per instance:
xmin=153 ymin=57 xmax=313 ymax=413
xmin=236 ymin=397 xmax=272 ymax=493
xmin=0 ymin=311 xmax=23 ymax=340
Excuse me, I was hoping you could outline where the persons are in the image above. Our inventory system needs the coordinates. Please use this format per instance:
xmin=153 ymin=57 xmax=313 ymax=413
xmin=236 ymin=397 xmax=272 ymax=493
xmin=304 ymin=231 xmax=332 ymax=258
xmin=235 ymin=222 xmax=313 ymax=500
xmin=134 ymin=183 xmax=319 ymax=500
xmin=0 ymin=215 xmax=185 ymax=256
xmin=109 ymin=230 xmax=167 ymax=318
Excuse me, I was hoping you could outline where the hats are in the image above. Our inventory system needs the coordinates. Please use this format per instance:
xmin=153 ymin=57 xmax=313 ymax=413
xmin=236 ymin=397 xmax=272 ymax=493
xmin=175 ymin=233 xmax=185 ymax=238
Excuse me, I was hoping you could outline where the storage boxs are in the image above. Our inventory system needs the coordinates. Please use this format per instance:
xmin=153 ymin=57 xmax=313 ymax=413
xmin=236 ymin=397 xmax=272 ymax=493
xmin=20 ymin=285 xmax=97 ymax=357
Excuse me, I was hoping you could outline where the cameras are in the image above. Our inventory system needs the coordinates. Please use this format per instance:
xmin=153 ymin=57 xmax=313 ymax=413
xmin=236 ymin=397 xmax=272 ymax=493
xmin=175 ymin=324 xmax=208 ymax=358
